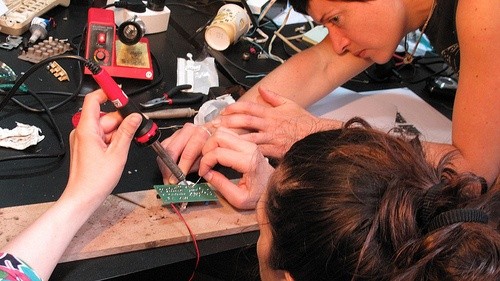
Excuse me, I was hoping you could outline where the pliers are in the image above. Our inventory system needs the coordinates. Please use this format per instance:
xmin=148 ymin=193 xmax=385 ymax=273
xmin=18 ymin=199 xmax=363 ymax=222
xmin=138 ymin=84 xmax=203 ymax=109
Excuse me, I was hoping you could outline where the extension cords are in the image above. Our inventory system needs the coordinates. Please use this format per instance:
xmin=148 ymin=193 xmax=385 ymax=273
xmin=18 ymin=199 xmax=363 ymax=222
xmin=108 ymin=0 xmax=169 ymax=36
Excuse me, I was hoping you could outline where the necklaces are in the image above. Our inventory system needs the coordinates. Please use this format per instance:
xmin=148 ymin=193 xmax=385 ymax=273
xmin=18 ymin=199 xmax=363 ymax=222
xmin=403 ymin=0 xmax=436 ymax=65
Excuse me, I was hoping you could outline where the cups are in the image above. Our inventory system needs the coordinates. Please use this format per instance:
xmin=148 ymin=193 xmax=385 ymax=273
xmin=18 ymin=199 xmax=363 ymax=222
xmin=204 ymin=3 xmax=251 ymax=51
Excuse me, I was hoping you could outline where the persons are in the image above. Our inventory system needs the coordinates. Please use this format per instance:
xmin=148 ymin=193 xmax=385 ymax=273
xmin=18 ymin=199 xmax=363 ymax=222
xmin=156 ymin=0 xmax=499 ymax=187
xmin=0 ymin=84 xmax=500 ymax=281
